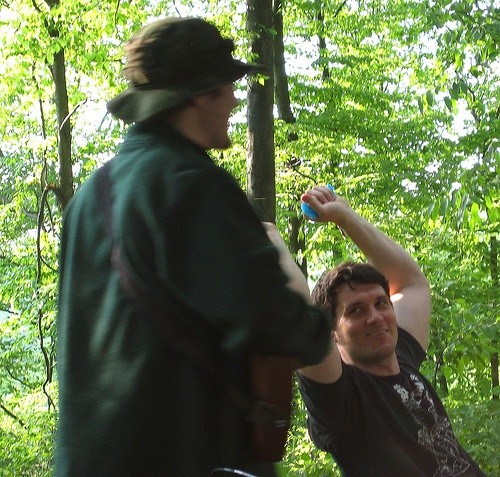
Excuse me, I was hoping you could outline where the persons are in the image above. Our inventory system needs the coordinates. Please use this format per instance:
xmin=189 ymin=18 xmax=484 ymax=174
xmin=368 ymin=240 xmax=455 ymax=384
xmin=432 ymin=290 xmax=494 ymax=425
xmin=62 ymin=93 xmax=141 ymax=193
xmin=56 ymin=16 xmax=333 ymax=477
xmin=262 ymin=184 xmax=492 ymax=477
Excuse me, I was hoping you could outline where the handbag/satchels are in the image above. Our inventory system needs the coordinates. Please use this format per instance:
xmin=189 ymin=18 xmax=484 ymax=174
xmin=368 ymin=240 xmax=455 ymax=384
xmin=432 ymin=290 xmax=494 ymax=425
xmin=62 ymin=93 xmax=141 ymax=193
xmin=249 ymin=353 xmax=295 ymax=461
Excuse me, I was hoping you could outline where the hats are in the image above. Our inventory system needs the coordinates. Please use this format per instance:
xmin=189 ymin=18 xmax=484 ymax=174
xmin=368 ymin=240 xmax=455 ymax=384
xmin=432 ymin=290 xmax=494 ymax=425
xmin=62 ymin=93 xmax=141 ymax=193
xmin=106 ymin=17 xmax=271 ymax=123
